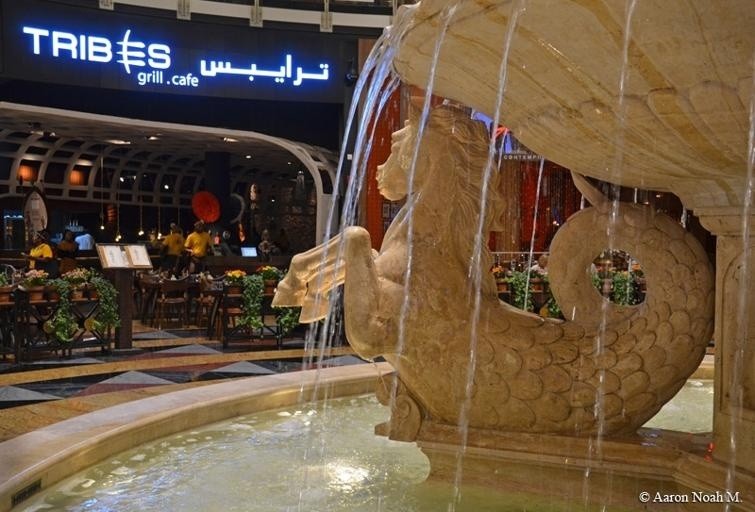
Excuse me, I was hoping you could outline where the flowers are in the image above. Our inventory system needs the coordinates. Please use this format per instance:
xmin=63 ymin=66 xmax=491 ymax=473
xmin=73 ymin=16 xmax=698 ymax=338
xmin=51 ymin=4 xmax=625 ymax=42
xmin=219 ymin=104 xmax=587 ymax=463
xmin=62 ymin=268 xmax=89 ymax=284
xmin=256 ymin=266 xmax=279 ymax=280
xmin=225 ymin=270 xmax=246 ymax=285
xmin=492 ymin=266 xmax=504 ymax=279
xmin=24 ymin=270 xmax=48 ymax=285
xmin=526 ymin=265 xmax=544 ymax=278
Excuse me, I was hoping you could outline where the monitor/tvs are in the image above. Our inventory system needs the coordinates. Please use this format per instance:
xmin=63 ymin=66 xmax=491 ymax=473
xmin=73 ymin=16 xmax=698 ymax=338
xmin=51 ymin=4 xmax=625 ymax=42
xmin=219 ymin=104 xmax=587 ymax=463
xmin=239 ymin=245 xmax=258 ymax=256
xmin=465 ymin=104 xmax=550 ymax=161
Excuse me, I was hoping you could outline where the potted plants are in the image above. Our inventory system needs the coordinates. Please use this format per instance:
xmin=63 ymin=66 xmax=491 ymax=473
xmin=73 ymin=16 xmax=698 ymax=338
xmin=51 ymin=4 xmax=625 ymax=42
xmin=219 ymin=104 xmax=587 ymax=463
xmin=0 ymin=273 xmax=12 ymax=302
xmin=47 ymin=278 xmax=75 ymax=345
xmin=88 ymin=276 xmax=122 ymax=328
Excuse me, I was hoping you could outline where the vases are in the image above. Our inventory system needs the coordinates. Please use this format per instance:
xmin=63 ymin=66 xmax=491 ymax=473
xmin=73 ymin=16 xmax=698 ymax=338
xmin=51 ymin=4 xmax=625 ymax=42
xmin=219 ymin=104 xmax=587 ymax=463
xmin=264 ymin=280 xmax=276 ymax=293
xmin=72 ymin=285 xmax=83 ymax=299
xmin=530 ymin=278 xmax=544 ymax=290
xmin=229 ymin=286 xmax=240 ymax=294
xmin=497 ymin=282 xmax=507 ymax=291
xmin=29 ymin=287 xmax=44 ymax=300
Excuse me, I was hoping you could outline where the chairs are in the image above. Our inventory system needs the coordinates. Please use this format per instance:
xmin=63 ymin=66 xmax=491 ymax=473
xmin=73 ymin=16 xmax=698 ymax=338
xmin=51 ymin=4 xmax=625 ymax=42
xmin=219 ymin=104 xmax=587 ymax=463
xmin=133 ymin=268 xmax=226 ymax=329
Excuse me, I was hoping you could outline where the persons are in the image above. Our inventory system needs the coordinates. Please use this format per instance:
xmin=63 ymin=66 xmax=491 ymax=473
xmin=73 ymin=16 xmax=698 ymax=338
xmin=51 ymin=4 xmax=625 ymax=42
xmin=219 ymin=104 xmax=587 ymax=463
xmin=159 ymin=222 xmax=186 ymax=266
xmin=257 ymin=229 xmax=275 ymax=256
xmin=57 ymin=229 xmax=78 ymax=253
xmin=21 ymin=229 xmax=60 ymax=279
xmin=75 ymin=229 xmax=95 ymax=250
xmin=183 ymin=221 xmax=216 ymax=273
xmin=529 ymin=254 xmax=548 ymax=277
